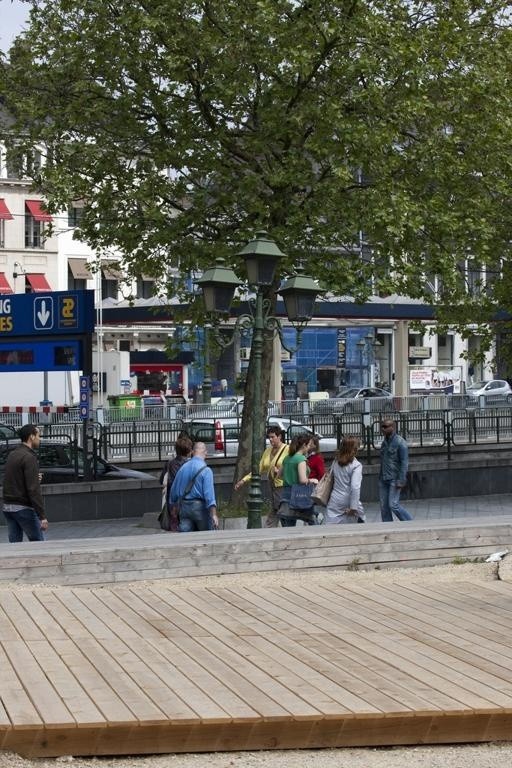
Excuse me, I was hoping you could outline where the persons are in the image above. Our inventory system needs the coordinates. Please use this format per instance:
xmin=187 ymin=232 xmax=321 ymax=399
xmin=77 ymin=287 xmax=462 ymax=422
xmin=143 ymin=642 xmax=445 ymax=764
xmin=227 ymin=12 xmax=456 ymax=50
xmin=158 ymin=376 xmax=168 ymax=395
xmin=322 ymin=434 xmax=365 ymax=524
xmin=278 ymin=432 xmax=320 ymax=527
xmin=377 ymin=419 xmax=413 ymax=522
xmin=1 ymin=423 xmax=48 ymax=543
xmin=444 ymin=379 xmax=453 ymax=395
xmin=299 ymin=434 xmax=325 ymax=527
xmin=159 ymin=435 xmax=194 ymax=532
xmin=362 ymin=391 xmax=369 ymax=397
xmin=470 ymin=372 xmax=475 ymax=385
xmin=433 ymin=370 xmax=460 ymax=386
xmin=234 ymin=425 xmax=291 ymax=527
xmin=167 ymin=440 xmax=219 ymax=532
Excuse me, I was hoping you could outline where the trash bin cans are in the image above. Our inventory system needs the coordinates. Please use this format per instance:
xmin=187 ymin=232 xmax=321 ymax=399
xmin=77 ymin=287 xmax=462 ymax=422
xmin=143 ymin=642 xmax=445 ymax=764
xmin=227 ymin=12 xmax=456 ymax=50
xmin=107 ymin=394 xmax=141 ymax=423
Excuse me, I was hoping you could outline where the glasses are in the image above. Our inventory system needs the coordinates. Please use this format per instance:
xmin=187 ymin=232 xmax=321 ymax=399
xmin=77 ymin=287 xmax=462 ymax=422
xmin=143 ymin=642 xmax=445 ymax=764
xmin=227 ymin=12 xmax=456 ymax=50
xmin=380 ymin=423 xmax=394 ymax=428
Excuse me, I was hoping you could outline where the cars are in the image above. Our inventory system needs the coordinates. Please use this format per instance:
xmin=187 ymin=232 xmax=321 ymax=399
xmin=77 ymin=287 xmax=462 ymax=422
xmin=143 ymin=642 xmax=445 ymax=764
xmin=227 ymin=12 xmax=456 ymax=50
xmin=185 ymin=396 xmax=281 ymax=423
xmin=0 ymin=421 xmax=48 ymax=450
xmin=0 ymin=439 xmax=158 ymax=486
xmin=311 ymin=385 xmax=399 ymax=417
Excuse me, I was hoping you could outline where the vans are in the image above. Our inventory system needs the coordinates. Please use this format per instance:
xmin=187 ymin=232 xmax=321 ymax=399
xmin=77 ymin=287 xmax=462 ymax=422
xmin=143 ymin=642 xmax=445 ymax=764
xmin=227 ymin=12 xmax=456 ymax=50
xmin=181 ymin=418 xmax=339 ymax=460
xmin=466 ymin=380 xmax=512 ymax=404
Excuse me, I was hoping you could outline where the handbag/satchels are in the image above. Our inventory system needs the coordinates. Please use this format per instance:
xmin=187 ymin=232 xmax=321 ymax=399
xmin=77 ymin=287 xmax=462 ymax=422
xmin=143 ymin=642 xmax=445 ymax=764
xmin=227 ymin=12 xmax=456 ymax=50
xmin=157 ymin=501 xmax=171 ymax=532
xmin=272 ymin=487 xmax=283 ymax=510
xmin=288 ymin=483 xmax=315 ymax=512
xmin=310 ymin=455 xmax=337 ymax=508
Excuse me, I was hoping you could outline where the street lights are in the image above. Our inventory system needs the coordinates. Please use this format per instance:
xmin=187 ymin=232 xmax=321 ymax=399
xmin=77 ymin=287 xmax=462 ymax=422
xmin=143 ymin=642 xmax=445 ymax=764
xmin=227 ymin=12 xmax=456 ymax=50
xmin=193 ymin=228 xmax=327 ymax=530
xmin=357 ymin=333 xmax=384 ymax=388
xmin=190 ymin=285 xmax=226 ymax=403
